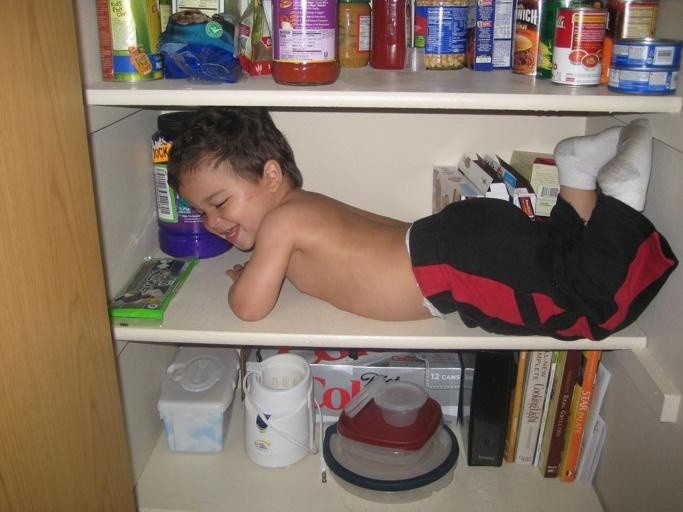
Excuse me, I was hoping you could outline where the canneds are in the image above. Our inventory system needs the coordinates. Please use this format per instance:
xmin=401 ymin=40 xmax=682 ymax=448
xmin=271 ymin=0 xmax=469 ymax=86
xmin=551 ymin=8 xmax=683 ymax=95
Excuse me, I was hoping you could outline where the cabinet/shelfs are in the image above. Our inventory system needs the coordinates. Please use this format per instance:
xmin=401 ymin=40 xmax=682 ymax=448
xmin=65 ymin=0 xmax=683 ymax=512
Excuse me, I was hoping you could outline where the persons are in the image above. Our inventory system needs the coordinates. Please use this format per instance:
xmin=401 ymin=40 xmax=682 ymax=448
xmin=166 ymin=105 xmax=680 ymax=344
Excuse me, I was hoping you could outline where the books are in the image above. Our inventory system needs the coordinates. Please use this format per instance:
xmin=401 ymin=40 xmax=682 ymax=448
xmin=501 ymin=351 xmax=613 ymax=484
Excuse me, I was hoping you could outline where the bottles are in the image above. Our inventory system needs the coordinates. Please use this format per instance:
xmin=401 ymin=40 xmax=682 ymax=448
xmin=149 ymin=111 xmax=235 ymax=261
